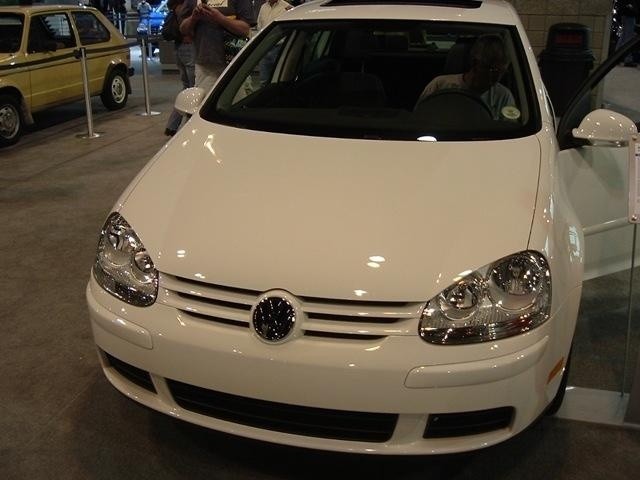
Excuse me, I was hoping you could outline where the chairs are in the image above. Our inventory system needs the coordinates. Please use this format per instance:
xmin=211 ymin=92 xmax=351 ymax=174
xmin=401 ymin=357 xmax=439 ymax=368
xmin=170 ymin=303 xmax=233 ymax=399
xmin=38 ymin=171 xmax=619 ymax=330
xmin=446 ymin=41 xmax=472 ymax=75
xmin=288 ymin=30 xmax=386 ymax=108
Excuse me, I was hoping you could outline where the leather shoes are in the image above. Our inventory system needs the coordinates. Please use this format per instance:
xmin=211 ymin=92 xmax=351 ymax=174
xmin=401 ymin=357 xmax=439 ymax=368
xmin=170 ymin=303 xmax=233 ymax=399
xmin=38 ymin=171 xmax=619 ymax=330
xmin=165 ymin=127 xmax=176 ymax=136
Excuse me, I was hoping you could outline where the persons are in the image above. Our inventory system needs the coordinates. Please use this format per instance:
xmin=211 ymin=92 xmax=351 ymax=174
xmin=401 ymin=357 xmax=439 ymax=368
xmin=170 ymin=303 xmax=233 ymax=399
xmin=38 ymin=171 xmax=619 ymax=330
xmin=416 ymin=33 xmax=519 ymax=124
xmin=90 ymin=1 xmax=300 ymax=136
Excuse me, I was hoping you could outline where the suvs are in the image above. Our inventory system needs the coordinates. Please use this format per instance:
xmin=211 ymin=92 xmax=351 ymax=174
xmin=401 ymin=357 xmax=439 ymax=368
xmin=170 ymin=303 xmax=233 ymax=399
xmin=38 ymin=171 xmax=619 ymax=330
xmin=0 ymin=3 xmax=135 ymax=146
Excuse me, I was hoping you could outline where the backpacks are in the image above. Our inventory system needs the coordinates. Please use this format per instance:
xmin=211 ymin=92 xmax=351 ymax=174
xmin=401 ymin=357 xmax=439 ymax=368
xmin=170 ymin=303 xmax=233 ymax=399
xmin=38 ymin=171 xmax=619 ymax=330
xmin=160 ymin=7 xmax=179 ymax=41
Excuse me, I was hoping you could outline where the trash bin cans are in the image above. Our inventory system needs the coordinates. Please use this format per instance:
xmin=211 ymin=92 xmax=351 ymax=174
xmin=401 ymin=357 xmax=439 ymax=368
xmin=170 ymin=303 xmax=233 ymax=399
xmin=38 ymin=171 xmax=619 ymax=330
xmin=538 ymin=23 xmax=594 ymax=117
